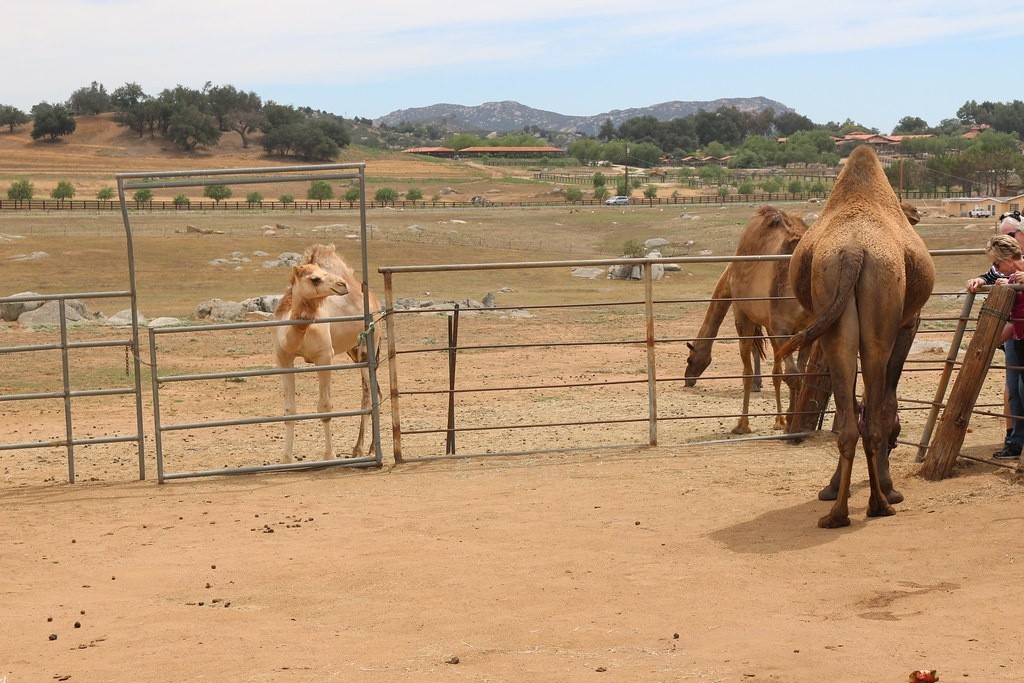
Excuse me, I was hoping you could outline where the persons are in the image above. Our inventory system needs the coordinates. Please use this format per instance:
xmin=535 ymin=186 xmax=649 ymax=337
xmin=966 ymin=212 xmax=1024 ymax=459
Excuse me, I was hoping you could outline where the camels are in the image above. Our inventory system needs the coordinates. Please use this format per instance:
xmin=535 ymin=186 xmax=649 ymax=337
xmin=685 ymin=201 xmax=812 ymax=431
xmin=773 ymin=146 xmax=938 ymax=528
xmin=269 ymin=243 xmax=382 ymax=463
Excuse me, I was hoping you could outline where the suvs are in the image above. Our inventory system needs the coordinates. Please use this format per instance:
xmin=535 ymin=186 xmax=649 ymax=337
xmin=605 ymin=196 xmax=628 ymax=205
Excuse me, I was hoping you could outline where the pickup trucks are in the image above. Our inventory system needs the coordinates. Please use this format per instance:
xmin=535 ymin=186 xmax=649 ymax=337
xmin=968 ymin=208 xmax=991 ymax=218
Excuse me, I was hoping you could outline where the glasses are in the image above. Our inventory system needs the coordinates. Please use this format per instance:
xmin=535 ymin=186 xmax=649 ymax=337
xmin=992 ymin=260 xmax=1000 ymax=268
xmin=1007 ymin=229 xmax=1020 ymax=238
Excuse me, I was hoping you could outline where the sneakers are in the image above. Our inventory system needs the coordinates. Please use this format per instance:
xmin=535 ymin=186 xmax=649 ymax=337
xmin=992 ymin=443 xmax=1022 ymax=459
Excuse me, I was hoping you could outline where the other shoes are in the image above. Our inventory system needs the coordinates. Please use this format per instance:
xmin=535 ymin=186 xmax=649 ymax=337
xmin=1004 ymin=436 xmax=1012 ymax=449
